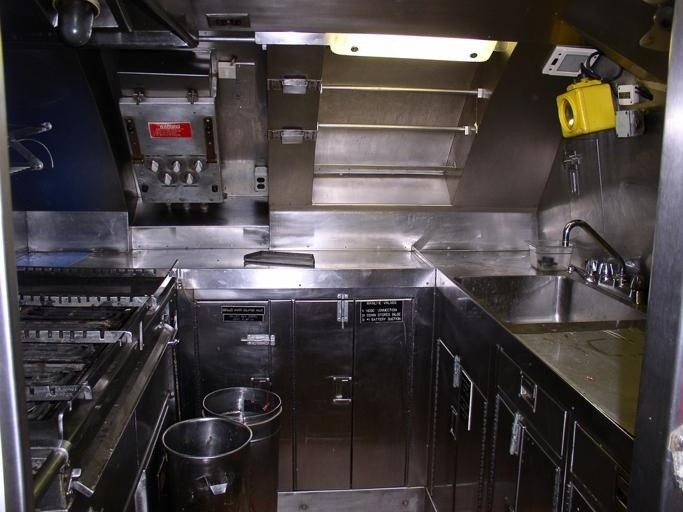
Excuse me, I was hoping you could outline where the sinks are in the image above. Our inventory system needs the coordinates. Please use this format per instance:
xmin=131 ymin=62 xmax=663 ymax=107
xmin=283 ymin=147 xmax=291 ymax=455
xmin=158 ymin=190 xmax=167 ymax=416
xmin=448 ymin=273 xmax=646 ymax=333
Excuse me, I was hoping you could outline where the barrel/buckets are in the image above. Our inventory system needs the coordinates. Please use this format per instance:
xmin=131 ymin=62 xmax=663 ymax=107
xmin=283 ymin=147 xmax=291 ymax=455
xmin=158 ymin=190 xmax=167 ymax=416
xmin=200 ymin=386 xmax=283 ymax=512
xmin=161 ymin=415 xmax=253 ymax=512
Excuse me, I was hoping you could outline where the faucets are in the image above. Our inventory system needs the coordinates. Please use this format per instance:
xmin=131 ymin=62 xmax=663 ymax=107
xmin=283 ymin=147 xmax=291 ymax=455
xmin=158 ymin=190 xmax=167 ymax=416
xmin=567 ymin=264 xmax=596 ymax=284
xmin=563 ymin=219 xmax=626 ymax=276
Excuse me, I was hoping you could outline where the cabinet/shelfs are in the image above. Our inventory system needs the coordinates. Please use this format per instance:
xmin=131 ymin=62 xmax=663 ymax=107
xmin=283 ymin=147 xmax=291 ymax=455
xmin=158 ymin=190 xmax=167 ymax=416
xmin=292 ymin=300 xmax=412 ymax=489
xmin=192 ymin=298 xmax=271 ymax=401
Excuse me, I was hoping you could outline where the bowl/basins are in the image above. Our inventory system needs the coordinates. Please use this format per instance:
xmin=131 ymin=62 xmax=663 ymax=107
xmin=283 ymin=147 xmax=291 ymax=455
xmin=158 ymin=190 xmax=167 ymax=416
xmin=524 ymin=238 xmax=574 ymax=272
